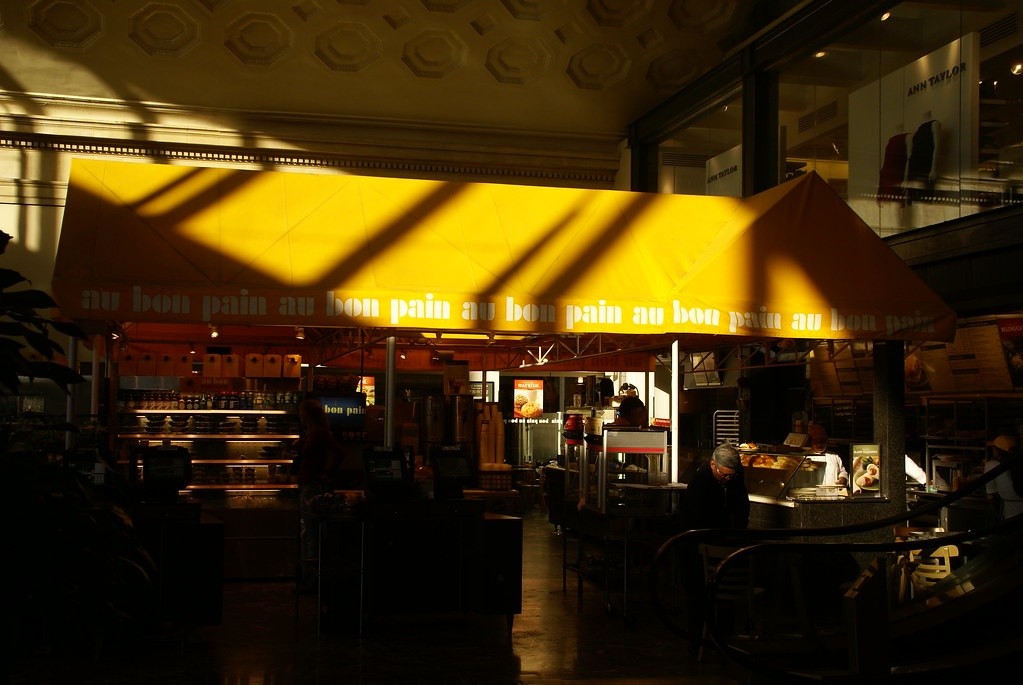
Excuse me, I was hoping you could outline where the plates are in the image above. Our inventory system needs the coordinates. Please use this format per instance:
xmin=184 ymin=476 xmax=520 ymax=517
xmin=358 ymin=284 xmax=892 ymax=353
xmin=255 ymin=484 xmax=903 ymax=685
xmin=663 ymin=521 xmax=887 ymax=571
xmin=816 ymin=485 xmax=845 ymax=489
xmin=855 ymin=470 xmax=879 ymax=491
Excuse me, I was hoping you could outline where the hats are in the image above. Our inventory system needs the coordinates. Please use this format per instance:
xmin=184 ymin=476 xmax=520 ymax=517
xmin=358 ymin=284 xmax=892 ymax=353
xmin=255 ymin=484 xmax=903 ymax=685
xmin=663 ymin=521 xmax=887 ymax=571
xmin=984 ymin=434 xmax=1017 ymax=454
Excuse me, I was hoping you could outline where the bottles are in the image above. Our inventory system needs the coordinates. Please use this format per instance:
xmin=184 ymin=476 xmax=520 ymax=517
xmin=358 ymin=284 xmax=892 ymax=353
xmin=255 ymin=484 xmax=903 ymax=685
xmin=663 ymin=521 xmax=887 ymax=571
xmin=115 ymin=389 xmax=301 ymax=485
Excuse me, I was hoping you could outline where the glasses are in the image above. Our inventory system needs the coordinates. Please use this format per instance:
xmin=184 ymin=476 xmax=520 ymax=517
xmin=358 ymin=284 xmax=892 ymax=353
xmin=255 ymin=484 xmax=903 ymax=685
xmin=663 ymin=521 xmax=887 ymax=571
xmin=715 ymin=467 xmax=737 ymax=478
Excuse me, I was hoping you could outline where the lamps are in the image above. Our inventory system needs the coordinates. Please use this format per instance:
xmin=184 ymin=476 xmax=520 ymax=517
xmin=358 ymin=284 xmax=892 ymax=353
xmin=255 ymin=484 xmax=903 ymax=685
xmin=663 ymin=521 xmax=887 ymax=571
xmin=295 ymin=326 xmax=305 ymax=340
xmin=431 ymin=350 xmax=440 ymax=360
xmin=401 ymin=350 xmax=408 ymax=359
xmin=434 ymin=332 xmax=443 ymax=344
xmin=111 ymin=329 xmax=120 ymax=340
xmin=208 ymin=324 xmax=219 ymax=339
xmin=777 ymin=338 xmax=795 ymax=349
xmin=367 ymin=350 xmax=376 ymax=357
xmin=488 ymin=333 xmax=496 ymax=343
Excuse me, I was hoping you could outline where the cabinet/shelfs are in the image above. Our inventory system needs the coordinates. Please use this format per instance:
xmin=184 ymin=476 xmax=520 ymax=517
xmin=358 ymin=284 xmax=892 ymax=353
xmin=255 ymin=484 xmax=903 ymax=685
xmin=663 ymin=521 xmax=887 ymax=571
xmin=810 ymin=392 xmax=1023 ymax=513
xmin=117 ymin=407 xmax=300 ymax=507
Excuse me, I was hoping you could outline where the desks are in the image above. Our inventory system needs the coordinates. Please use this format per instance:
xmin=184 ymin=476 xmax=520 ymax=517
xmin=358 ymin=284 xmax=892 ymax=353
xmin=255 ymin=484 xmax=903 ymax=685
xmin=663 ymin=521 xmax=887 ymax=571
xmin=322 ymin=510 xmax=522 ymax=635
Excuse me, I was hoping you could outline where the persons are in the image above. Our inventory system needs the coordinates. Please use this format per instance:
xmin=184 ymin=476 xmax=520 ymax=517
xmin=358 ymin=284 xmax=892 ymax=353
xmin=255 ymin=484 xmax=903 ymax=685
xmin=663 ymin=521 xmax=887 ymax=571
xmin=983 ymin=435 xmax=1023 ymax=548
xmin=613 ymin=396 xmax=648 ymax=467
xmin=878 ymin=124 xmax=913 ymax=192
xmin=806 ymin=425 xmax=849 ymax=496
xmin=909 ymin=111 xmax=941 ymax=190
xmin=675 ymin=444 xmax=749 ymax=655
xmin=291 ymin=400 xmax=337 ymax=596
